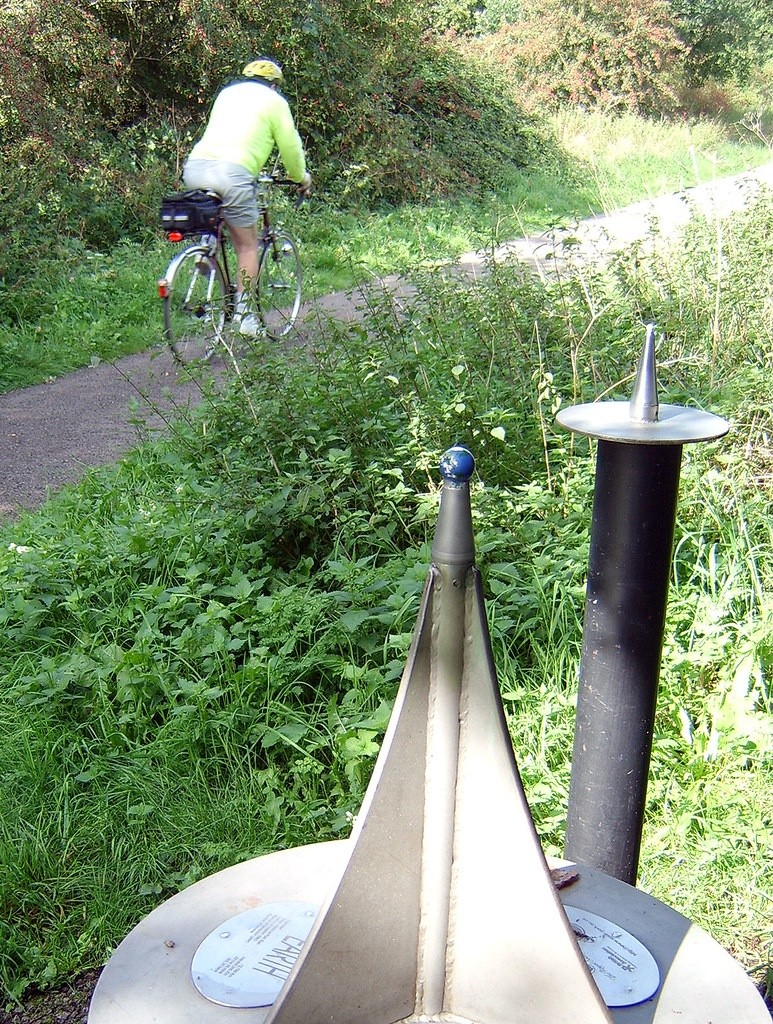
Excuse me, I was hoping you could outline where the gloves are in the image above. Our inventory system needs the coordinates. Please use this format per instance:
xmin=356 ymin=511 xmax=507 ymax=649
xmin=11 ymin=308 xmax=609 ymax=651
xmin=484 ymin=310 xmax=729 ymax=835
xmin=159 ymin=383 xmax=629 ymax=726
xmin=287 ymin=173 xmax=312 ymax=197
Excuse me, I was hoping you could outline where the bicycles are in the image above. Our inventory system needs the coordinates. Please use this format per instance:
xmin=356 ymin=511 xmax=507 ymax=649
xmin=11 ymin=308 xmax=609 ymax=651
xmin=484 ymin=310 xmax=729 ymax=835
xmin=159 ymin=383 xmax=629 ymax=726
xmin=158 ymin=170 xmax=318 ymax=368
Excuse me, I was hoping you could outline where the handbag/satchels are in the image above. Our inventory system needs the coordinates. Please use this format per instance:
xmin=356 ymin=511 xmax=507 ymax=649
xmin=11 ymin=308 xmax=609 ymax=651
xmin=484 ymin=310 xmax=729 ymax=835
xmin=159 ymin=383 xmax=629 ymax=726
xmin=160 ymin=186 xmax=223 ymax=233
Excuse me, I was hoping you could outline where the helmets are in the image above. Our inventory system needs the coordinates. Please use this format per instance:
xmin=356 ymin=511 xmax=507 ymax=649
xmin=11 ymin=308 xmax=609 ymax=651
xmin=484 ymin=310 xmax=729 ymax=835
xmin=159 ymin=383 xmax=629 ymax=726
xmin=242 ymin=60 xmax=284 ymax=82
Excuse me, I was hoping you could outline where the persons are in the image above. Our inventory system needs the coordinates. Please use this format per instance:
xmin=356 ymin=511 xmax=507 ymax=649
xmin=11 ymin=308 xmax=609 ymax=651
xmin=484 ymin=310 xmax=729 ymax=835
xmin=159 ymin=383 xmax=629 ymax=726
xmin=182 ymin=60 xmax=311 ymax=339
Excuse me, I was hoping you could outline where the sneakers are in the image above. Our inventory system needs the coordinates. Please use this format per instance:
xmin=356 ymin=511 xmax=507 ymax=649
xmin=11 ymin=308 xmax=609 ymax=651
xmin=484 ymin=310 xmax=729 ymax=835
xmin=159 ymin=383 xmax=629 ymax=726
xmin=230 ymin=312 xmax=267 ymax=338
xmin=195 ymin=252 xmax=217 ymax=278
